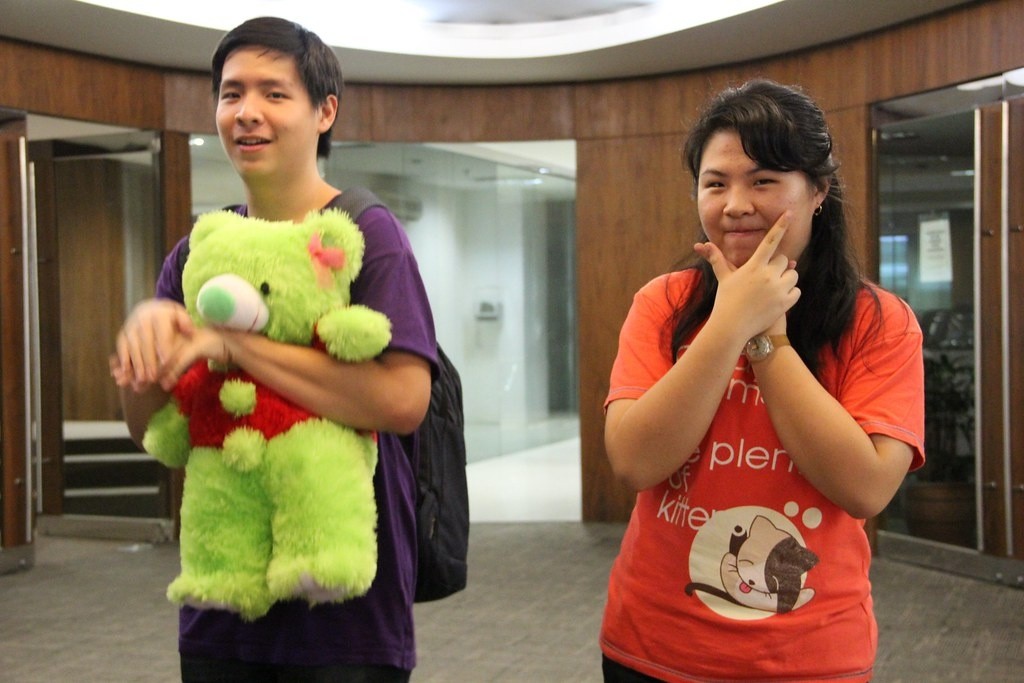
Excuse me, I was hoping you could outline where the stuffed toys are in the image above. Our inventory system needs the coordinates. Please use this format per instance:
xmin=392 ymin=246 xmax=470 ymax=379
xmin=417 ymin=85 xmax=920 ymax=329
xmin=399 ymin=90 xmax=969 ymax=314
xmin=139 ymin=205 xmax=393 ymax=621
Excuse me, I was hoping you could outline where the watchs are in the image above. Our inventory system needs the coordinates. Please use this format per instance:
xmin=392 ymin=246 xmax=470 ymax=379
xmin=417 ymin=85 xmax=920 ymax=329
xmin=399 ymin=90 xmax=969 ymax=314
xmin=744 ymin=333 xmax=790 ymax=363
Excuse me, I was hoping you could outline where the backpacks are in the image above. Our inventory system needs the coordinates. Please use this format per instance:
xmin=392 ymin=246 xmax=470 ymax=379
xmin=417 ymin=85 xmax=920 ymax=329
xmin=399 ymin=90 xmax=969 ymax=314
xmin=176 ymin=186 xmax=470 ymax=603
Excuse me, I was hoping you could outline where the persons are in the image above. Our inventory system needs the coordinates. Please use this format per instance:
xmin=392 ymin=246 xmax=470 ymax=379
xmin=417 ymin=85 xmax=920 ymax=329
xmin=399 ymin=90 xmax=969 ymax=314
xmin=104 ymin=15 xmax=442 ymax=683
xmin=594 ymin=81 xmax=925 ymax=683
